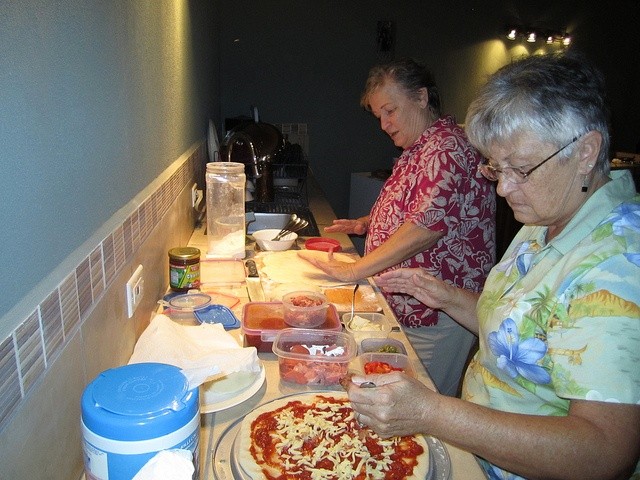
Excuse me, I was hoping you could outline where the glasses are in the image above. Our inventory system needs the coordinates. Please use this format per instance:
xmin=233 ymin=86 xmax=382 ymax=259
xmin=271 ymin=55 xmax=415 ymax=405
xmin=476 ymin=134 xmax=583 ymax=184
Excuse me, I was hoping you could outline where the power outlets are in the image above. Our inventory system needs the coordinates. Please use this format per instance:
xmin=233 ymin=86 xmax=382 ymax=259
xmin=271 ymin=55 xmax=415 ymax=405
xmin=127 ymin=264 xmax=145 ymax=319
xmin=191 ymin=182 xmax=199 ymax=208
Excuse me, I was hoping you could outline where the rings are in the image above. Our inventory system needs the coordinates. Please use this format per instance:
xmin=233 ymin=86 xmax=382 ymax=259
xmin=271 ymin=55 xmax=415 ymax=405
xmin=357 ymin=414 xmax=367 ymax=428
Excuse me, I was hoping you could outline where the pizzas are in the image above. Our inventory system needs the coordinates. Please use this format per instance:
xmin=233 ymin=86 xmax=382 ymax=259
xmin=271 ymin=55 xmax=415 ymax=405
xmin=235 ymin=392 xmax=433 ymax=480
xmin=168 ymin=245 xmax=200 ymax=291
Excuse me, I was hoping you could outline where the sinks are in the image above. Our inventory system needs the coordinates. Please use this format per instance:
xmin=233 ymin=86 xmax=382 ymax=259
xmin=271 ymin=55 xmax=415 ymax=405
xmin=246 ymin=211 xmax=317 ymax=236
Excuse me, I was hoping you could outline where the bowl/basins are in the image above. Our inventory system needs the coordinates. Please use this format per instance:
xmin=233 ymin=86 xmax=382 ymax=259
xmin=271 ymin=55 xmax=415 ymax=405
xmin=247 ymin=180 xmax=254 ymax=193
xmin=252 ymin=229 xmax=298 ymax=250
xmin=246 ymin=190 xmax=253 ymax=202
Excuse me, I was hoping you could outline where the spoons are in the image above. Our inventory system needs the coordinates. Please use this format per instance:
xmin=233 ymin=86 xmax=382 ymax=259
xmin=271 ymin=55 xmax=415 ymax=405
xmin=347 ymin=284 xmax=359 ymax=328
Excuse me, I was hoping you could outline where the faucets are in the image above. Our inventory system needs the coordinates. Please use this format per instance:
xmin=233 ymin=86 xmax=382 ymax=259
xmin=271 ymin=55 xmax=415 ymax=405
xmin=224 ymin=133 xmax=263 ymax=179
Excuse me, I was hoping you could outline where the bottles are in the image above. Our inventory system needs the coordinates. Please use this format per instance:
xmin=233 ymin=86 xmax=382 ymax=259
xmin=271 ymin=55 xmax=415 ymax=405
xmin=206 ymin=161 xmax=247 ymax=262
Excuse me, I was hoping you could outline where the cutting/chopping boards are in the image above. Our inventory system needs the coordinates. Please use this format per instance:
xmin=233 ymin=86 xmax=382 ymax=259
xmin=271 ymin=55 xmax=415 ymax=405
xmin=243 ymin=251 xmax=382 ymax=313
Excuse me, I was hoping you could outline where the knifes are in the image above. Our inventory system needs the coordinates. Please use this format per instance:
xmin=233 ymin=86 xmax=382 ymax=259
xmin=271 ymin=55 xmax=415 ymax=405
xmin=244 ymin=260 xmax=266 ymax=301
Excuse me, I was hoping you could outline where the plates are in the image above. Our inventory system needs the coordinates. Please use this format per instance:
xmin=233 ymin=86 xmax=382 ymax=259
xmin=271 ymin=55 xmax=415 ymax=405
xmin=198 ymin=356 xmax=265 ymax=413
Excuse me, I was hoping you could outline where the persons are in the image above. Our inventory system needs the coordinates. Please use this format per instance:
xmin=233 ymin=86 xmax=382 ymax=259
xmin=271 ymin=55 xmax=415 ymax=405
xmin=347 ymin=54 xmax=640 ymax=479
xmin=299 ymin=57 xmax=496 ymax=394
